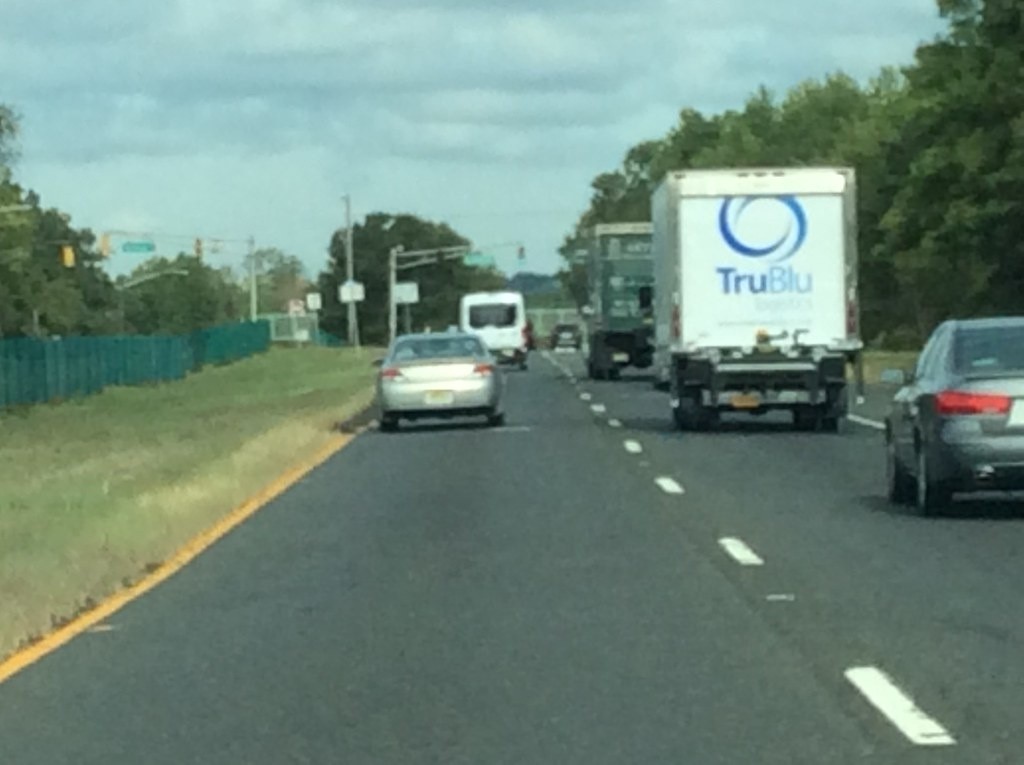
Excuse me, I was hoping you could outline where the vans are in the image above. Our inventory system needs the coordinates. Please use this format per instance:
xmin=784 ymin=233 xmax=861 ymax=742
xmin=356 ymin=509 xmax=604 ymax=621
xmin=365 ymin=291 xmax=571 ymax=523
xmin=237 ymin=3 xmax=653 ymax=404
xmin=459 ymin=290 xmax=529 ymax=370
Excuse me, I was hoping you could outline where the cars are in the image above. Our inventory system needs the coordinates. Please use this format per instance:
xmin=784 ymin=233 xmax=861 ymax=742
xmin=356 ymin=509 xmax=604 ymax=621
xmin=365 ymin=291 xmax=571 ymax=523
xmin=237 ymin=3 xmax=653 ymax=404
xmin=549 ymin=324 xmax=582 ymax=349
xmin=373 ymin=332 xmax=502 ymax=431
xmin=887 ymin=316 xmax=1024 ymax=513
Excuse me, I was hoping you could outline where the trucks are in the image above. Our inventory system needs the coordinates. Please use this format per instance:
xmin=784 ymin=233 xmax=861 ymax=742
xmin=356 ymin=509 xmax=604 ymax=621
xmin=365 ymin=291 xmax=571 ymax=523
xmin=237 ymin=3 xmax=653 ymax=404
xmin=587 ymin=218 xmax=656 ymax=381
xmin=649 ymin=166 xmax=864 ymax=435
